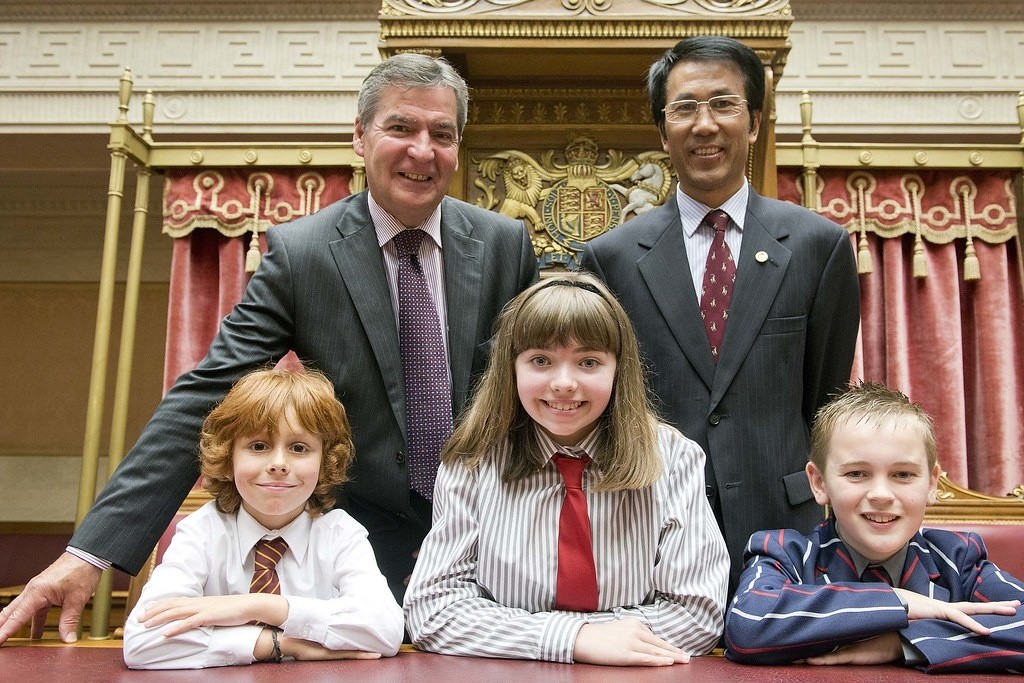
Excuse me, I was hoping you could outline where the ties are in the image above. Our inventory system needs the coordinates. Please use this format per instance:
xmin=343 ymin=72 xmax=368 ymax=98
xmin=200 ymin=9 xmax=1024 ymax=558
xmin=861 ymin=567 xmax=892 ymax=587
xmin=700 ymin=209 xmax=736 ymax=365
xmin=392 ymin=228 xmax=453 ymax=504
xmin=551 ymin=452 xmax=598 ymax=611
xmin=246 ymin=536 xmax=288 ymax=629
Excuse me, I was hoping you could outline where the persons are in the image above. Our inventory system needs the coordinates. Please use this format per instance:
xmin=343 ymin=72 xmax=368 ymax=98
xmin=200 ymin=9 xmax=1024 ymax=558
xmin=576 ymin=35 xmax=861 ymax=647
xmin=123 ymin=363 xmax=405 ymax=671
xmin=722 ymin=378 xmax=1024 ymax=673
xmin=403 ymin=273 xmax=731 ymax=667
xmin=0 ymin=55 xmax=539 ymax=649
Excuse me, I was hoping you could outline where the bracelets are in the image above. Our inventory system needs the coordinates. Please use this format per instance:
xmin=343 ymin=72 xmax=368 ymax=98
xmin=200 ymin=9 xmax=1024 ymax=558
xmin=264 ymin=627 xmax=284 ymax=663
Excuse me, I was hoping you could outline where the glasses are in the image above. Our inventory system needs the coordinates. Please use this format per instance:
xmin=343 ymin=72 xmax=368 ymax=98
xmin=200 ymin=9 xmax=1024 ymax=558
xmin=660 ymin=94 xmax=753 ymax=121
